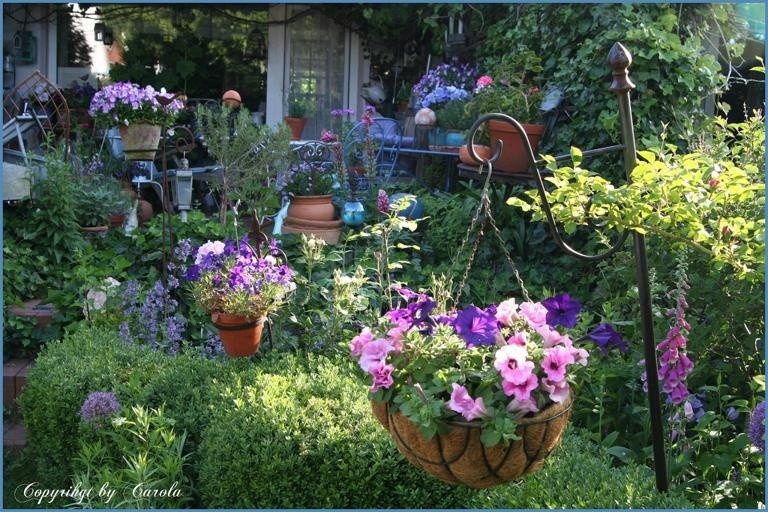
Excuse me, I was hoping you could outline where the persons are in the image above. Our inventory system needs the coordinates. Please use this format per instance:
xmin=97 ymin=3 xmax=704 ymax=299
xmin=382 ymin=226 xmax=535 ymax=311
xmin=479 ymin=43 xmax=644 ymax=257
xmin=155 ymin=127 xmax=219 ymax=217
xmin=166 ymin=91 xmax=205 ymax=142
xmin=216 ymin=89 xmax=251 ymax=147
xmin=122 ymin=184 xmax=162 ymax=222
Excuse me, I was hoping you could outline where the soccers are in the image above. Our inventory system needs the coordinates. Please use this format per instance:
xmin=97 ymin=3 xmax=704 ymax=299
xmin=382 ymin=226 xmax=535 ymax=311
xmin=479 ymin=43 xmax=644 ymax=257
xmin=223 ymin=91 xmax=241 ymax=109
xmin=416 ymin=107 xmax=436 ymax=130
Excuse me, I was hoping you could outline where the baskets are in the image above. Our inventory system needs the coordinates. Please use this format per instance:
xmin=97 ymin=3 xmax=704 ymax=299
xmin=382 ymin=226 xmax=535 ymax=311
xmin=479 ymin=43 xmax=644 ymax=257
xmin=363 ymin=384 xmax=577 ymax=491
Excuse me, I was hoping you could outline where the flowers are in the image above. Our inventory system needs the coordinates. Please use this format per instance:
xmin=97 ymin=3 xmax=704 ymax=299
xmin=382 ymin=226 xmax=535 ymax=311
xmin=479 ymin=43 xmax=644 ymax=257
xmin=99 ymin=18 xmax=588 ymax=488
